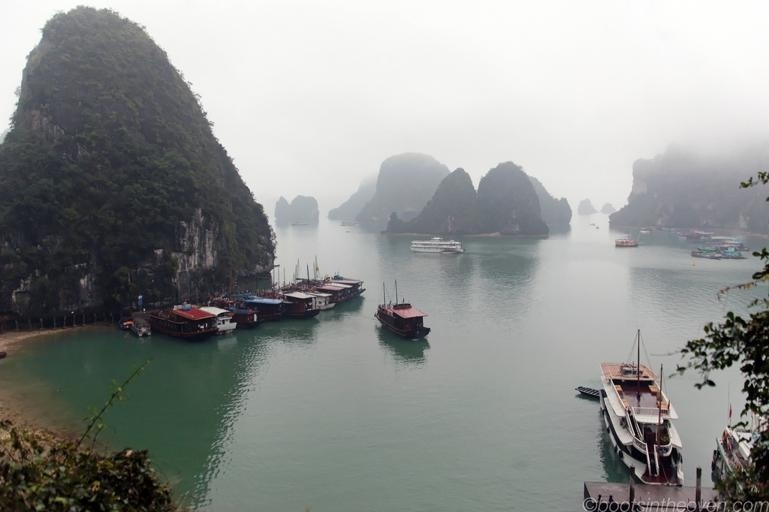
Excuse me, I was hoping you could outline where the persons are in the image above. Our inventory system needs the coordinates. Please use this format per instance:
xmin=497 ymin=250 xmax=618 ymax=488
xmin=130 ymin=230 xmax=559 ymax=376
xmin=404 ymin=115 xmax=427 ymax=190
xmin=645 ymin=427 xmax=656 ymax=476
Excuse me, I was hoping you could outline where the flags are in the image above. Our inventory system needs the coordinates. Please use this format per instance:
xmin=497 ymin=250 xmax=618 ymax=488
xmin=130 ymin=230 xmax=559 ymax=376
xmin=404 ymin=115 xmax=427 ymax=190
xmin=728 ymin=405 xmax=732 ymax=419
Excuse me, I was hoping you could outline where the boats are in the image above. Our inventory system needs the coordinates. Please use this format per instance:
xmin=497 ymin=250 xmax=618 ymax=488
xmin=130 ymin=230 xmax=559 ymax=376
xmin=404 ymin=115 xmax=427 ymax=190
xmin=408 ymin=235 xmax=465 ymax=256
xmin=291 ymin=220 xmax=308 ymax=226
xmin=615 ymin=238 xmax=639 ymax=248
xmin=672 ymin=228 xmax=751 ymax=259
xmin=574 ymin=385 xmax=605 ymax=399
xmin=639 ymin=225 xmax=657 ymax=234
xmin=120 ymin=255 xmax=368 ymax=350
xmin=710 ymin=380 xmax=769 ymax=486
xmin=373 ymin=279 xmax=431 ymax=341
xmin=600 ymin=326 xmax=689 ymax=486
